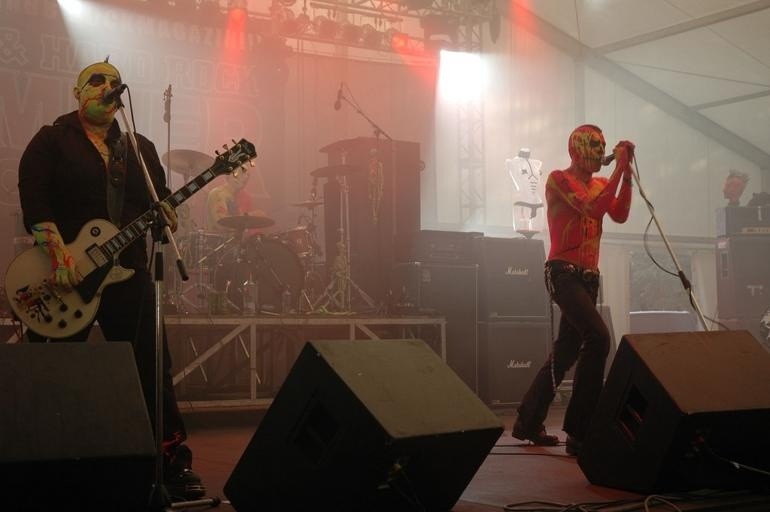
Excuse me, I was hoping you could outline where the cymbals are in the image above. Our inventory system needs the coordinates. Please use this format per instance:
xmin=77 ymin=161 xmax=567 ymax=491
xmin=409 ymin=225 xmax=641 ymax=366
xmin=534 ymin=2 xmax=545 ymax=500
xmin=291 ymin=202 xmax=323 ymax=207
xmin=218 ymin=215 xmax=272 ymax=229
xmin=161 ymin=149 xmax=214 ymax=177
xmin=311 ymin=164 xmax=370 ymax=176
xmin=319 ymin=138 xmax=363 ymax=153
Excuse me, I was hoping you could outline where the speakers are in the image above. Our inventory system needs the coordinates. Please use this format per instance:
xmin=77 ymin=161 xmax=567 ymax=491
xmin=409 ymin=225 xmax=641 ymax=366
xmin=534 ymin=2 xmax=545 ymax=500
xmin=327 ymin=140 xmax=420 ymax=313
xmin=577 ymin=330 xmax=770 ymax=497
xmin=396 ymin=264 xmax=479 ymax=400
xmin=479 ymin=323 xmax=555 ymax=407
xmin=715 ymin=237 xmax=770 ymax=319
xmin=0 ymin=340 xmax=157 ymax=512
xmin=479 ymin=238 xmax=550 ymax=322
xmin=720 ymin=319 xmax=770 ymax=353
xmin=224 ymin=339 xmax=505 ymax=512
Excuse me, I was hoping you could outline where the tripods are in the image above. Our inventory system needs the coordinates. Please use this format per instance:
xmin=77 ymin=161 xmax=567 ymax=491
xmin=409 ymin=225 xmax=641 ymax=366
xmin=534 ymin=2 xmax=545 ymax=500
xmin=142 ymin=227 xmax=220 ymax=512
xmin=311 ymin=180 xmax=376 ymax=316
xmin=162 ymin=236 xmax=242 ymax=313
xmin=350 ymin=135 xmax=404 ymax=318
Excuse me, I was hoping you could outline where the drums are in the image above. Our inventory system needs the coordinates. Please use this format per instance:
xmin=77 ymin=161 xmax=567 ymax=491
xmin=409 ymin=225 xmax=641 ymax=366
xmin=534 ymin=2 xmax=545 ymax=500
xmin=236 ymin=228 xmax=304 ymax=314
xmin=279 ymin=226 xmax=310 ymax=259
xmin=183 ymin=232 xmax=226 ymax=269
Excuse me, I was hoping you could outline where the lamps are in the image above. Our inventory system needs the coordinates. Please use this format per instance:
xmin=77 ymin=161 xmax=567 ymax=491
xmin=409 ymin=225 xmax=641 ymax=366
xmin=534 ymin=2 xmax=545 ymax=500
xmin=166 ymin=0 xmax=413 ymax=53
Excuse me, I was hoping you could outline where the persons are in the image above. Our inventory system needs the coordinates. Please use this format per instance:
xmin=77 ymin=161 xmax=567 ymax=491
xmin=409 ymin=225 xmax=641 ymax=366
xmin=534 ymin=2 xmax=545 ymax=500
xmin=511 ymin=122 xmax=634 ymax=458
xmin=495 ymin=147 xmax=546 ymax=233
xmin=16 ymin=61 xmax=208 ymax=500
xmin=208 ymin=163 xmax=253 ymax=238
xmin=722 ymin=170 xmax=750 ymax=207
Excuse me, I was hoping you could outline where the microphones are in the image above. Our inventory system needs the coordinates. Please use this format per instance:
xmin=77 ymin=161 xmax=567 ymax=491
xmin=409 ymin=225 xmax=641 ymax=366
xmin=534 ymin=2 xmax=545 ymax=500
xmin=104 ymin=84 xmax=126 ymax=104
xmin=256 ymin=235 xmax=265 ymax=249
xmin=334 ymin=89 xmax=343 ymax=110
xmin=163 ymin=100 xmax=171 ymax=123
xmin=601 ymin=153 xmax=616 ymax=166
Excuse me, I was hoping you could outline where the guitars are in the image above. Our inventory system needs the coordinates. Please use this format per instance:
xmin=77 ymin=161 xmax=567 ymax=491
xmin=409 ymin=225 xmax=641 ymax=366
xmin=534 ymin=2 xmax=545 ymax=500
xmin=4 ymin=138 xmax=258 ymax=339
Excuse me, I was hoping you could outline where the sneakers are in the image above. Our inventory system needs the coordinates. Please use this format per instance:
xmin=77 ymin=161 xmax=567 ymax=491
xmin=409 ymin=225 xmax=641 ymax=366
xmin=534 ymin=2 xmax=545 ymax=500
xmin=512 ymin=413 xmax=559 ymax=446
xmin=566 ymin=434 xmax=580 ymax=454
xmin=166 ymin=467 xmax=206 ymax=498
